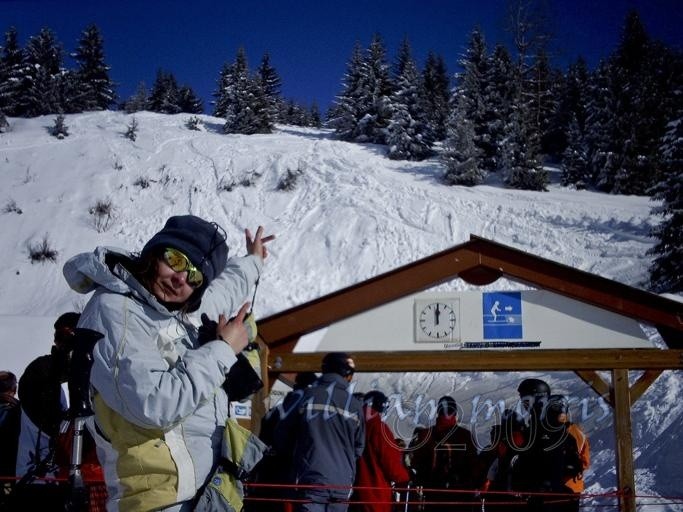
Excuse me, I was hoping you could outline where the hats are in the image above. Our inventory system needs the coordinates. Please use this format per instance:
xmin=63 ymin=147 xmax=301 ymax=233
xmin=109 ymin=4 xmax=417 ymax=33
xmin=139 ymin=214 xmax=230 ymax=288
xmin=321 ymin=351 xmax=356 ymax=378
xmin=437 ymin=395 xmax=458 ymax=418
xmin=352 ymin=391 xmax=391 ymax=413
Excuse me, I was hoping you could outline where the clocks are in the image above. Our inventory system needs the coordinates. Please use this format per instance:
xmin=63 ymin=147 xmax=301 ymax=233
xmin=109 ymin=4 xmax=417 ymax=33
xmin=413 ymin=298 xmax=460 ymax=344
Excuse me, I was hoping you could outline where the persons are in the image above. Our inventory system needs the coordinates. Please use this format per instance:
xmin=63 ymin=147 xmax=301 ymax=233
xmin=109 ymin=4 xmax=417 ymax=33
xmin=0 ymin=216 xmax=266 ymax=512
xmin=409 ymin=378 xmax=591 ymax=512
xmin=242 ymin=352 xmax=410 ymax=512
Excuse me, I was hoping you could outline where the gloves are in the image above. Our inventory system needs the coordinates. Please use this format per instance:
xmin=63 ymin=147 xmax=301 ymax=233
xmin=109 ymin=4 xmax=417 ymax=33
xmin=197 ymin=313 xmax=263 ymax=402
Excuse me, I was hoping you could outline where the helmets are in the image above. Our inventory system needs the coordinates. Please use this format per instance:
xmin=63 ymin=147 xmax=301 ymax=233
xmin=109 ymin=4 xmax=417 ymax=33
xmin=518 ymin=378 xmax=550 ymax=412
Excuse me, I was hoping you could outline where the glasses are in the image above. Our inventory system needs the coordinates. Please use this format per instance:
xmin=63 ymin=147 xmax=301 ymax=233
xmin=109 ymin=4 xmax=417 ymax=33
xmin=158 ymin=245 xmax=203 ymax=290
